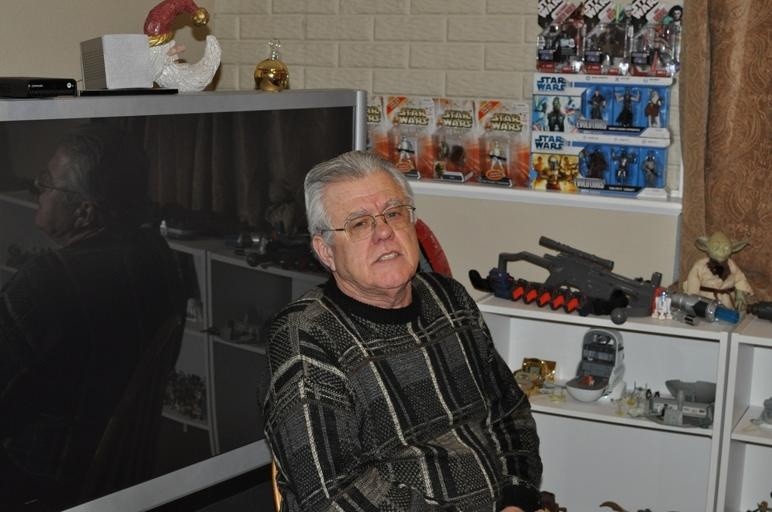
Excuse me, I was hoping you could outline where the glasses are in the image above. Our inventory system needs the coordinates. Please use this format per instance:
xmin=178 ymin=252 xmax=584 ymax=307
xmin=34 ymin=171 xmax=77 ymax=194
xmin=322 ymin=204 xmax=416 ymax=242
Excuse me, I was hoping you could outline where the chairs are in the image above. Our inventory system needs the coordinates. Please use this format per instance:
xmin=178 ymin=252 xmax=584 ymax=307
xmin=84 ymin=317 xmax=183 ymax=491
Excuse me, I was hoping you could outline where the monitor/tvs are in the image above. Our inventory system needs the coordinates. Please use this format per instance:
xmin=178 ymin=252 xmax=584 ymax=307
xmin=1 ymin=86 xmax=369 ymax=511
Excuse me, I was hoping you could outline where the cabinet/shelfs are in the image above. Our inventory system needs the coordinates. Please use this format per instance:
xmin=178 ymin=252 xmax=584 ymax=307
xmin=161 ymin=230 xmax=224 ymax=455
xmin=207 ymin=243 xmax=328 ymax=454
xmin=1 ymin=194 xmax=38 ymax=285
xmin=716 ymin=313 xmax=771 ymax=511
xmin=474 ymin=294 xmax=745 ymax=511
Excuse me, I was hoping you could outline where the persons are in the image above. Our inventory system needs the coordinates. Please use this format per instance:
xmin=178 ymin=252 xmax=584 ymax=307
xmin=534 ymin=18 xmax=671 ymax=194
xmin=261 ymin=152 xmax=543 ymax=512
xmin=684 ymin=230 xmax=753 ymax=311
xmin=0 ymin=131 xmax=190 ymax=511
